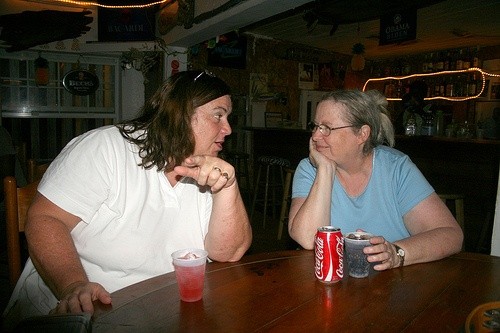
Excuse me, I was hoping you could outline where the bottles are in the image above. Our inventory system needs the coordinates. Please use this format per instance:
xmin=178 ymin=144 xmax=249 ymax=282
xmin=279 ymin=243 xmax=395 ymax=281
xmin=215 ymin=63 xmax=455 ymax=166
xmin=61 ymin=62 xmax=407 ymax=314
xmin=385 ymin=78 xmax=411 ymax=98
xmin=424 ymin=76 xmax=440 ymax=98
xmin=422 ymin=52 xmax=444 ymax=74
xmin=463 ymin=47 xmax=482 ymax=70
xmin=478 ymin=111 xmax=494 ymax=139
xmin=447 ymin=120 xmax=475 ymax=139
xmin=334 ymin=57 xmax=347 ymax=80
xmin=368 ymin=55 xmax=415 ymax=76
xmin=400 ymin=111 xmax=443 ymax=136
xmin=440 ymin=74 xmax=454 ymax=97
xmin=445 ymin=49 xmax=463 ymax=71
xmin=454 ymin=72 xmax=487 ymax=97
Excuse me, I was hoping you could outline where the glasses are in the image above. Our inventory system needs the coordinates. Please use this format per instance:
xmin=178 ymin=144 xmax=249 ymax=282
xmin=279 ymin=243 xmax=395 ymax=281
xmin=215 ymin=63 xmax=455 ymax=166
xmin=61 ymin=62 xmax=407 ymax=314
xmin=194 ymin=69 xmax=218 ymax=81
xmin=308 ymin=121 xmax=361 ymax=137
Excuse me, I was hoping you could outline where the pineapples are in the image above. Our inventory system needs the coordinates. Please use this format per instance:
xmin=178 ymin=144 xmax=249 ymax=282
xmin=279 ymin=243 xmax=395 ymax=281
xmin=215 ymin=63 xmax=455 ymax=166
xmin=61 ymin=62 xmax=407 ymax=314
xmin=351 ymin=42 xmax=365 ymax=71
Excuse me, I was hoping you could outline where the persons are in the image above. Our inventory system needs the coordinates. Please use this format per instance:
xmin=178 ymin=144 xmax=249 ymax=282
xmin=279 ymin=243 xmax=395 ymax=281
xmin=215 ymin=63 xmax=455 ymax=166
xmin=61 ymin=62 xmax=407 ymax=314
xmin=288 ymin=89 xmax=464 ymax=270
xmin=393 ymin=81 xmax=437 ymax=135
xmin=3 ymin=71 xmax=253 ymax=333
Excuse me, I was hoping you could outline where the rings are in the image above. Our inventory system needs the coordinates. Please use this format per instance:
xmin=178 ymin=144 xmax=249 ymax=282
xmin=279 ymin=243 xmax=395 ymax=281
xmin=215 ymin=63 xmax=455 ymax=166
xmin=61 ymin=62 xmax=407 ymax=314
xmin=215 ymin=168 xmax=221 ymax=172
xmin=57 ymin=300 xmax=62 ymax=304
xmin=222 ymin=173 xmax=228 ymax=178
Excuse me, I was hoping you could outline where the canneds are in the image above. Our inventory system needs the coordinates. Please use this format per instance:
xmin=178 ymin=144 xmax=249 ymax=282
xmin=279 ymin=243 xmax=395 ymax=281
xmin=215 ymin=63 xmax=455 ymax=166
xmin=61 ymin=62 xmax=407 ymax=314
xmin=314 ymin=226 xmax=344 ymax=284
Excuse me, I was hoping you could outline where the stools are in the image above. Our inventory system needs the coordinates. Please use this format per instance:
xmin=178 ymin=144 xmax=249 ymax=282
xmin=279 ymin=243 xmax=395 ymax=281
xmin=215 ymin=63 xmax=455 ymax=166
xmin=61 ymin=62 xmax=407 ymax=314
xmin=435 ymin=189 xmax=466 ymax=232
xmin=224 ymin=152 xmax=297 ymax=241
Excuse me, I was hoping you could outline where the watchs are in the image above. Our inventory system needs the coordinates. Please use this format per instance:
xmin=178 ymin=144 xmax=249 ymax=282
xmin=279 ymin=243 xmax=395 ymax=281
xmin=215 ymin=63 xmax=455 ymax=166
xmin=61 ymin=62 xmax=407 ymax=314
xmin=393 ymin=244 xmax=405 ymax=267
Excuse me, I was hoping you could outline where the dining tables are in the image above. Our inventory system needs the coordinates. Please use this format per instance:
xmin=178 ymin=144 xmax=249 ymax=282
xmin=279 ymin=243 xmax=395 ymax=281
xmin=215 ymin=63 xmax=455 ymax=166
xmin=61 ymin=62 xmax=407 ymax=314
xmin=66 ymin=249 xmax=500 ymax=333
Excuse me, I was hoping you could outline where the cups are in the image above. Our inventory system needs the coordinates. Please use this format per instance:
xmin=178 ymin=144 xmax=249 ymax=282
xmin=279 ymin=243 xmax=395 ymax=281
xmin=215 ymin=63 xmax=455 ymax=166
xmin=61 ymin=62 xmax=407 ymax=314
xmin=172 ymin=249 xmax=208 ymax=302
xmin=344 ymin=232 xmax=373 ymax=278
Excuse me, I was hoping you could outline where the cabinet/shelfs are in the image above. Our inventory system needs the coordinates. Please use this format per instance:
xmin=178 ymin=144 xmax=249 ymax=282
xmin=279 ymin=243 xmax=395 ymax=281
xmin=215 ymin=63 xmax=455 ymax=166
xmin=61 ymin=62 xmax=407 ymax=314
xmin=346 ymin=27 xmax=500 ymax=124
